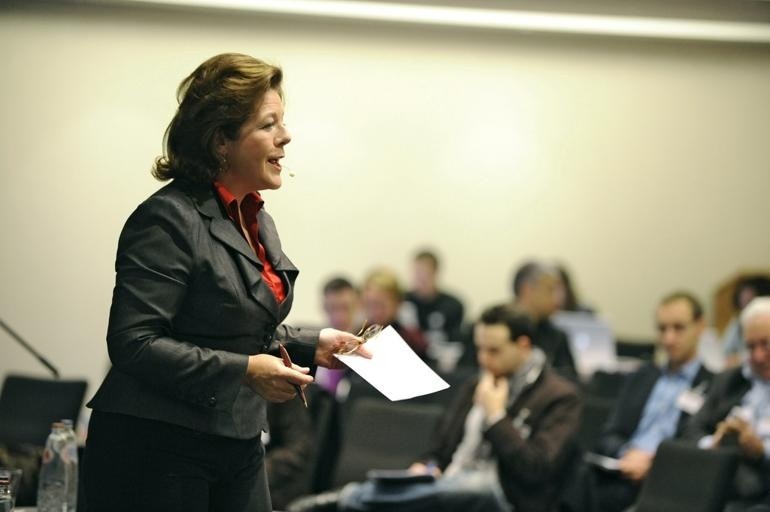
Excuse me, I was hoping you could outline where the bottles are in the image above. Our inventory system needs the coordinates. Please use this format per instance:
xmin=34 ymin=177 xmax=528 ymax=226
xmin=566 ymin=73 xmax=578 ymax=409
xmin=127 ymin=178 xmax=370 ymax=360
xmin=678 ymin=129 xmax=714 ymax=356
xmin=35 ymin=418 xmax=81 ymax=512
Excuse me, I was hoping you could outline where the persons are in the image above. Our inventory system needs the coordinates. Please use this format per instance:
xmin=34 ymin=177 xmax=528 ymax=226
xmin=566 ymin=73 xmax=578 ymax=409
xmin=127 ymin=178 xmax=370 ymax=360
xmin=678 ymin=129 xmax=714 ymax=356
xmin=74 ymin=53 xmax=377 ymax=511
xmin=284 ymin=252 xmax=770 ymax=511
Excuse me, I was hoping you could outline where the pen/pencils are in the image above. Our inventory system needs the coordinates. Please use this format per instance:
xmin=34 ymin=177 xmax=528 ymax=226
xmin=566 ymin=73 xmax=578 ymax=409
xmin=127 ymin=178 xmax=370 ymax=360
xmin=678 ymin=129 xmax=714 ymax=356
xmin=279 ymin=344 xmax=308 ymax=408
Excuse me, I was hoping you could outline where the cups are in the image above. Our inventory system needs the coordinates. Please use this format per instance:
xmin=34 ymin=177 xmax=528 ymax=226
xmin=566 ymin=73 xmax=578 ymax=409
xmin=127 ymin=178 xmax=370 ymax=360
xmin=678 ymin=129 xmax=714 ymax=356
xmin=0 ymin=467 xmax=24 ymax=512
